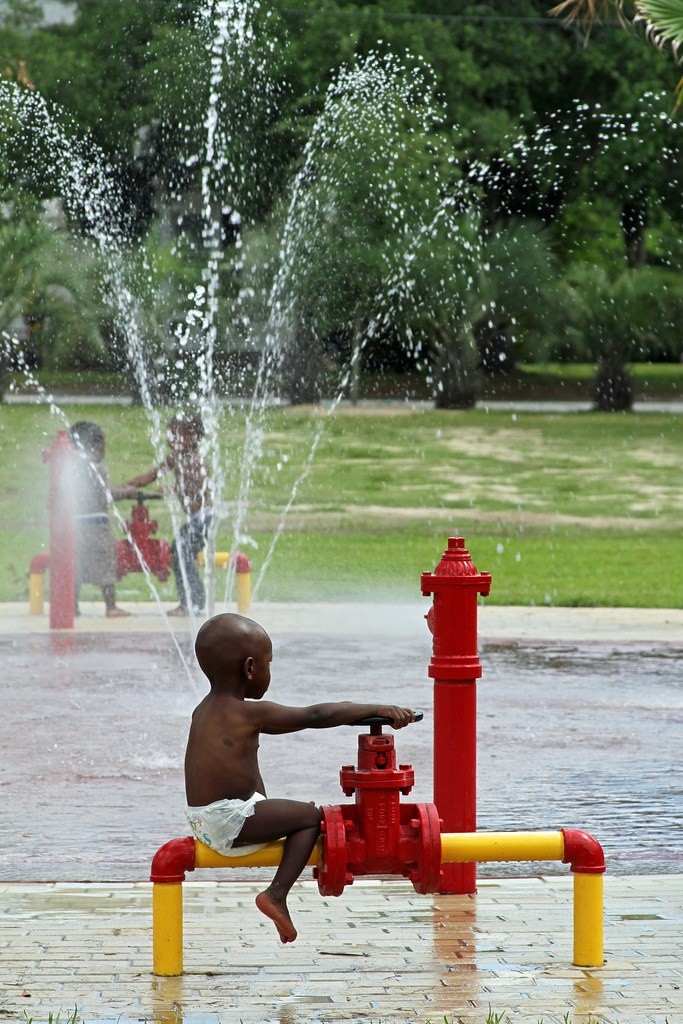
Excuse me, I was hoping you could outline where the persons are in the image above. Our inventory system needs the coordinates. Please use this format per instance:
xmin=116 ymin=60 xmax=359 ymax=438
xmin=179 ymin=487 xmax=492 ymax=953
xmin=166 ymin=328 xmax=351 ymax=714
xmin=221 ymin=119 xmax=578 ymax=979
xmin=116 ymin=410 xmax=211 ymax=616
xmin=70 ymin=421 xmax=137 ymax=617
xmin=185 ymin=612 xmax=415 ymax=944
